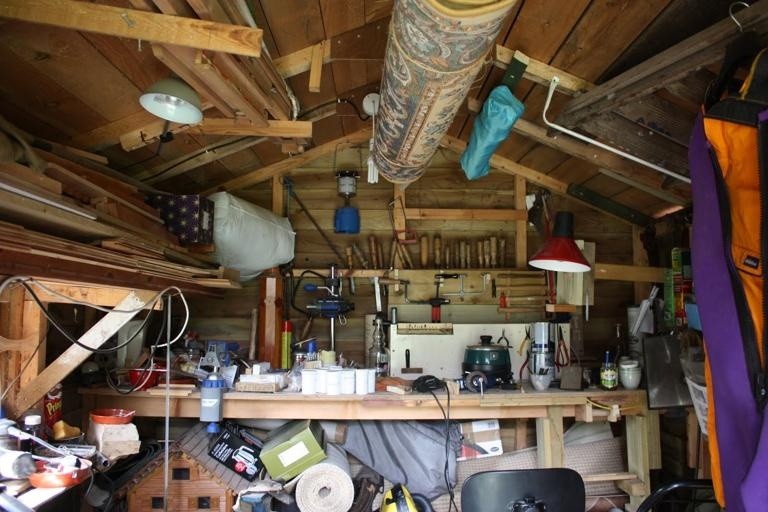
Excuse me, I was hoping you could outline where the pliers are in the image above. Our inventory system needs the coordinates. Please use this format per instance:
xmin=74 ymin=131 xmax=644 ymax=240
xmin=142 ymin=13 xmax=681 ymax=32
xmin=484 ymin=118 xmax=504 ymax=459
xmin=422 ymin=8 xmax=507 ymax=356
xmin=518 ymin=325 xmax=535 ymax=355
xmin=519 ymin=350 xmax=532 ymax=379
xmin=495 ymin=327 xmax=510 ymax=346
xmin=556 ymin=326 xmax=570 ymax=367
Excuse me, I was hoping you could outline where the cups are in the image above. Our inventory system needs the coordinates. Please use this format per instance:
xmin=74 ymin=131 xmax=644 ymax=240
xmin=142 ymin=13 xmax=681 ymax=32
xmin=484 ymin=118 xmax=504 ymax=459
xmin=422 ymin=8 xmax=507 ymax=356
xmin=321 ymin=350 xmax=337 ymax=366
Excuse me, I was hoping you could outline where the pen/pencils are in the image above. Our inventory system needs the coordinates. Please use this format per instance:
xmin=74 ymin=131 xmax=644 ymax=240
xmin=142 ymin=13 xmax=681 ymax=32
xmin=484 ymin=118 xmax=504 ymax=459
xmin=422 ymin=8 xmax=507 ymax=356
xmin=527 ymin=367 xmax=552 ymax=374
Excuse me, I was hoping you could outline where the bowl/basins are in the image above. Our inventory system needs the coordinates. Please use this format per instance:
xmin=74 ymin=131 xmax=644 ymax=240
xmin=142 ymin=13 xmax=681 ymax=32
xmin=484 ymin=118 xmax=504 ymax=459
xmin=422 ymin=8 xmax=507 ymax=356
xmin=130 ymin=368 xmax=161 ymax=389
xmin=619 ymin=360 xmax=642 ymax=390
xmin=26 ymin=458 xmax=92 ymax=488
xmin=530 ymin=373 xmax=552 ymax=391
xmin=49 ymin=431 xmax=86 ymax=444
xmin=89 ymin=407 xmax=134 ymax=425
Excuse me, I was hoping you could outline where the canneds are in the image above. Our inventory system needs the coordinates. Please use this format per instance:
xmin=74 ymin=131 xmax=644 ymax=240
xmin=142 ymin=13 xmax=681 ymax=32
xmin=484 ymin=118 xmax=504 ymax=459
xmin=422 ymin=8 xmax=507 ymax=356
xmin=600 ymin=362 xmax=619 ymax=391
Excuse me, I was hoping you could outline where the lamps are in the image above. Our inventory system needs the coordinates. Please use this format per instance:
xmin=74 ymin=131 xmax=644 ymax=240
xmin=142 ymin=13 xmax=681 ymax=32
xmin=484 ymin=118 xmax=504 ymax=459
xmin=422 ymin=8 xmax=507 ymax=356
xmin=362 ymin=93 xmax=380 ymax=184
xmin=139 ymin=71 xmax=204 ymax=124
xmin=333 ymin=169 xmax=360 ymax=234
xmin=528 ymin=186 xmax=592 ymax=273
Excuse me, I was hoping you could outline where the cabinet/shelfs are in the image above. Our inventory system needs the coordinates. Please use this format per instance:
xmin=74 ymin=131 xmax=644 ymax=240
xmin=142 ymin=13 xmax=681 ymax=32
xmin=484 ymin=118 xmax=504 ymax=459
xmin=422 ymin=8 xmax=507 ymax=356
xmin=76 ymin=380 xmax=648 ymax=512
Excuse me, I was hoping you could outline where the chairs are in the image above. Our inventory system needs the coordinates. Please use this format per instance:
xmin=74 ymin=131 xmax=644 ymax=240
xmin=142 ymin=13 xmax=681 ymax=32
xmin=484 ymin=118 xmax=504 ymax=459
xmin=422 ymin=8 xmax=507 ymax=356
xmin=637 ymin=479 xmax=712 ymax=511
xmin=460 ymin=469 xmax=586 ymax=511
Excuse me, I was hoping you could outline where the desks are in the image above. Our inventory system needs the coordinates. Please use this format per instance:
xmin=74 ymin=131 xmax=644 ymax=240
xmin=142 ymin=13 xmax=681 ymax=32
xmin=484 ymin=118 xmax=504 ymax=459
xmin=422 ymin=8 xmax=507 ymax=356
xmin=0 ymin=469 xmax=98 ymax=510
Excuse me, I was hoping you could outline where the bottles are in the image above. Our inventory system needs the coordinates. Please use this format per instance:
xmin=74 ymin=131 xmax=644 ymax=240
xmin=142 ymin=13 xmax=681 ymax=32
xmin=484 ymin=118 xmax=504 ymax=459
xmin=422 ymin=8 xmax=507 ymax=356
xmin=305 ymin=341 xmax=317 ymax=361
xmin=280 ymin=321 xmax=294 ymax=370
xmin=294 ymin=342 xmax=305 ymax=367
xmin=368 ymin=318 xmax=390 ymax=379
xmin=187 ymin=330 xmax=201 ymax=362
xmin=0 ymin=418 xmax=18 ymax=450
xmin=599 ymin=344 xmax=620 ymax=392
xmin=613 ymin=323 xmax=627 ymax=358
xmin=21 ymin=415 xmax=48 ymax=456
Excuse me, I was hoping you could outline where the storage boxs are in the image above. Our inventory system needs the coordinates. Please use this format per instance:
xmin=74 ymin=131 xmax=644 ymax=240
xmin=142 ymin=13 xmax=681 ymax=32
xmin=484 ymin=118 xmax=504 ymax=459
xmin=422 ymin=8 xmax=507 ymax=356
xmin=207 ymin=417 xmax=265 ymax=482
xmin=685 ymin=302 xmax=702 ymax=331
xmin=146 ymin=193 xmax=214 ymax=247
xmin=259 ymin=419 xmax=327 ymax=484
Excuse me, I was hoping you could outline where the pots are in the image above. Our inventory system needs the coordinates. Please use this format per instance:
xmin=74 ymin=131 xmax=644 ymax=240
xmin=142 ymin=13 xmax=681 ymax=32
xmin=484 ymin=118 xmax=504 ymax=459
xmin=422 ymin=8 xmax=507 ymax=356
xmin=462 ymin=335 xmax=512 ymax=388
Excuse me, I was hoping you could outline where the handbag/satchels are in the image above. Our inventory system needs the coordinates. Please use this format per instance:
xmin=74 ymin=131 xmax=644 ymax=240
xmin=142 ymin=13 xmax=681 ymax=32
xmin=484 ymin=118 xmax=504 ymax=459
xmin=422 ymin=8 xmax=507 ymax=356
xmin=342 ymin=418 xmax=462 ymax=500
